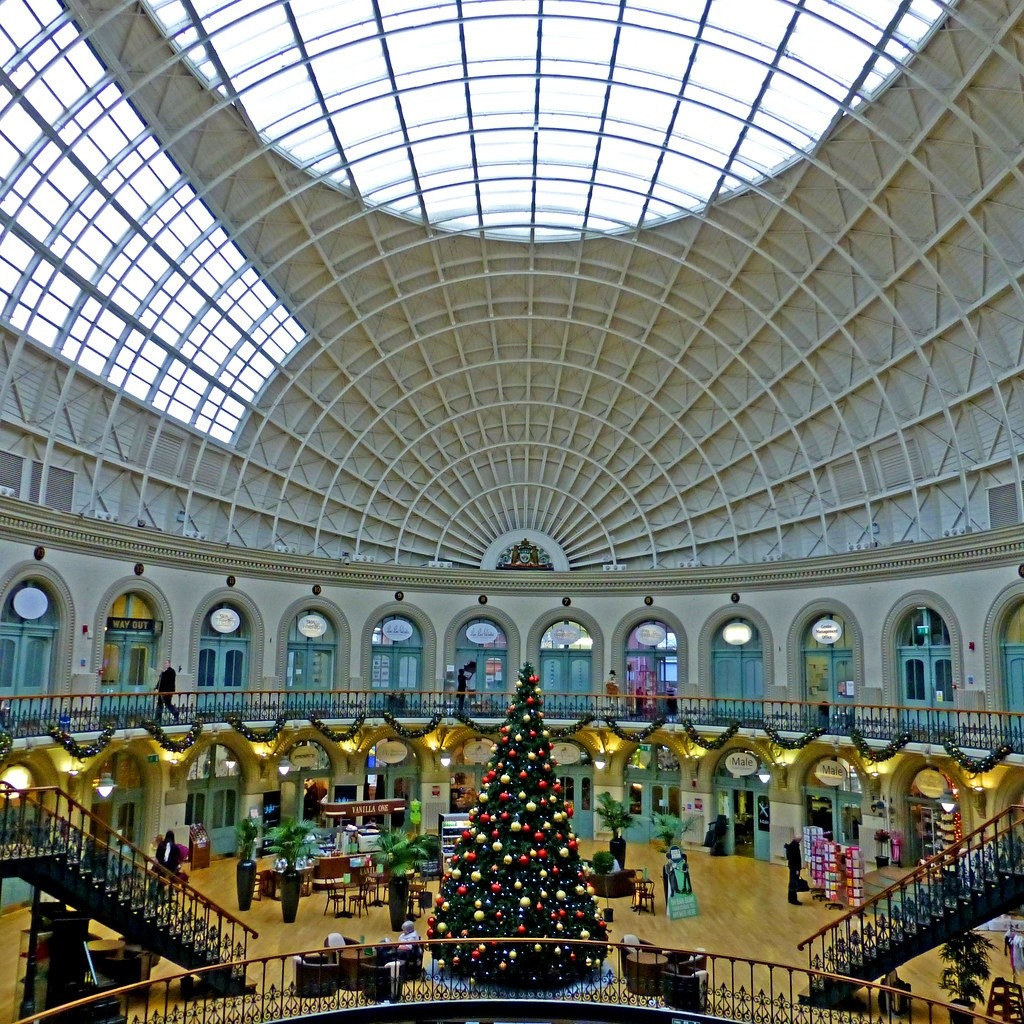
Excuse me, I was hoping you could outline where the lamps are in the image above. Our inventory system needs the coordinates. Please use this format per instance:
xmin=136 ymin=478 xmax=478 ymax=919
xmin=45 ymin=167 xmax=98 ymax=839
xmin=939 ymin=788 xmax=958 ymax=812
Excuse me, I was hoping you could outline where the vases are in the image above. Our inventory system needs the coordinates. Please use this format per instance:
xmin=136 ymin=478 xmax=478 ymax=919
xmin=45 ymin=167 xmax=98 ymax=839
xmin=419 ymin=890 xmax=433 ymax=909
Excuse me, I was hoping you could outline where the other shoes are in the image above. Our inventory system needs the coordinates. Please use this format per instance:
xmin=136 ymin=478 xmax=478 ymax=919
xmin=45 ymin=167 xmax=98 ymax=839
xmin=791 ymin=901 xmax=802 ymax=905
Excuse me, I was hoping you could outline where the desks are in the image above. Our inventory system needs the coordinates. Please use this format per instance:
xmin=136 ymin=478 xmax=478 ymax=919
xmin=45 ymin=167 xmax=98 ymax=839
xmin=635 ymin=877 xmax=652 ymax=884
xmin=626 ymin=952 xmax=668 ymax=996
xmin=341 ymin=949 xmax=373 ymax=991
xmin=366 ymin=873 xmax=389 ymax=907
xmin=408 ymin=884 xmax=426 ymax=918
xmin=87 ymin=939 xmax=125 ymax=959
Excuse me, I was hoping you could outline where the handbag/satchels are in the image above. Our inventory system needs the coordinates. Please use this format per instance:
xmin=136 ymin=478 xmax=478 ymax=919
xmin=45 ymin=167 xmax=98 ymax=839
xmin=795 ymin=876 xmax=810 ymax=891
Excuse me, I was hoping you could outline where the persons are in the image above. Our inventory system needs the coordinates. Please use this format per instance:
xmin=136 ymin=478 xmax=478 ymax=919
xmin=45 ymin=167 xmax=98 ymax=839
xmin=456 ymin=669 xmax=473 ymax=710
xmin=606 ymin=670 xmax=617 ymax=684
xmin=784 ymin=834 xmax=804 ymax=905
xmin=608 ymin=859 xmax=621 ymax=873
xmin=666 ymin=853 xmax=693 ymax=897
xmin=155 ymin=831 xmax=180 ymax=905
xmin=153 ymin=660 xmax=179 ymax=724
xmin=370 ymin=818 xmax=375 ymax=824
xmin=384 ymin=920 xmax=419 ymax=968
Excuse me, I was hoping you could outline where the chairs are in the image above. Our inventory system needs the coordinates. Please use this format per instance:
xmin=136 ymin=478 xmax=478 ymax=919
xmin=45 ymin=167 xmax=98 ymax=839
xmin=324 ymin=875 xmax=344 ymax=916
xmin=632 ymin=869 xmax=656 ymax=917
xmin=708 ymin=815 xmax=727 ymax=831
xmin=324 ymin=937 xmax=360 ymax=947
xmin=358 ymin=867 xmax=376 ymax=909
xmin=349 ymin=882 xmax=369 ymax=918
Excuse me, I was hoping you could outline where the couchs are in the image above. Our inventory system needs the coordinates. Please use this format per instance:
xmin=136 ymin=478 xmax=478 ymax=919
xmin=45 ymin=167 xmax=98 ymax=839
xmin=661 ymin=966 xmax=709 ymax=1014
xmin=662 ymin=949 xmax=708 ymax=970
xmin=375 ymin=944 xmax=424 ymax=981
xmin=588 ymin=868 xmax=637 ymax=898
xmin=292 ymin=955 xmax=338 ymax=998
xmin=620 ymin=938 xmax=654 ymax=977
xmin=118 ymin=938 xmax=160 ymax=968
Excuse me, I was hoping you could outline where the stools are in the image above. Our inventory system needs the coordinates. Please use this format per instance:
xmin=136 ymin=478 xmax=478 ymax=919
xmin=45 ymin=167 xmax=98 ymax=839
xmin=985 ymin=977 xmax=1024 ymax=1024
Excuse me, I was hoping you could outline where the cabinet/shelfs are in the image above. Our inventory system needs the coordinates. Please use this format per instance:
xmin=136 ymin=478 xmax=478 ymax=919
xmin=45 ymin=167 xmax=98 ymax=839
xmin=190 ymin=825 xmax=211 ymax=870
xmin=234 ymin=813 xmax=264 ymax=911
xmin=10 ymin=929 xmax=52 ymax=1023
xmin=920 ymin=806 xmax=940 ymax=857
xmin=437 ymin=813 xmax=470 ymax=865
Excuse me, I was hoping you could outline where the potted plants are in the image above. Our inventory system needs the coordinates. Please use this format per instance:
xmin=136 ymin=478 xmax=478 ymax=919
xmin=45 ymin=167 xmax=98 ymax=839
xmin=264 ymin=816 xmax=323 ymax=924
xmin=873 ymin=828 xmax=890 ymax=868
xmin=591 ymin=851 xmax=615 ymax=922
xmin=938 ymin=929 xmax=994 ymax=1024
xmin=366 ymin=828 xmax=441 ymax=932
xmin=592 ymin=791 xmax=635 ymax=871
